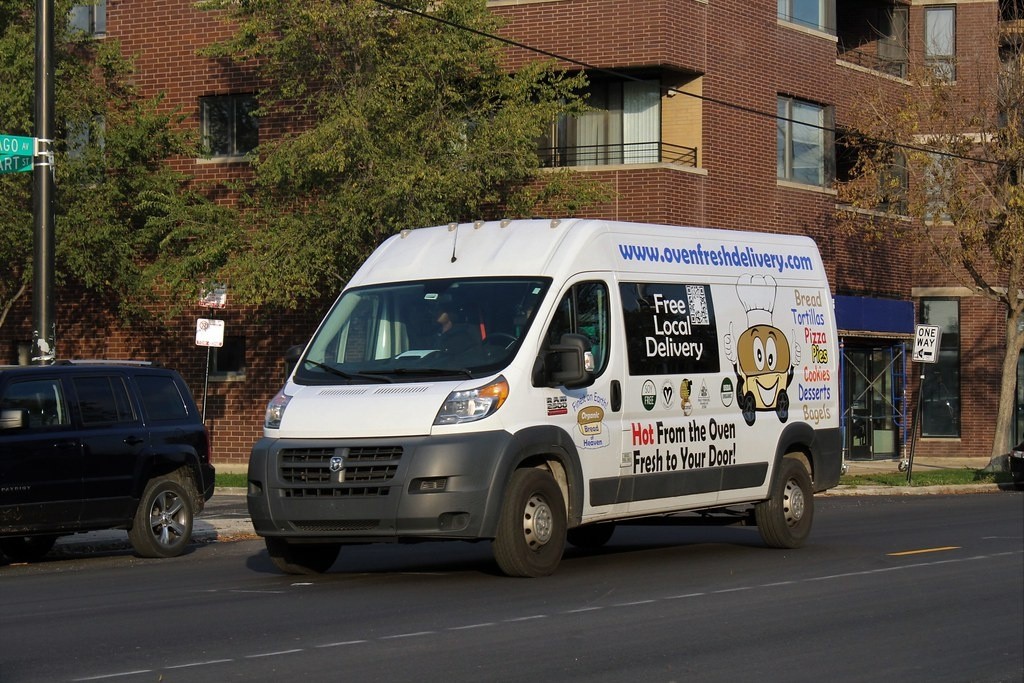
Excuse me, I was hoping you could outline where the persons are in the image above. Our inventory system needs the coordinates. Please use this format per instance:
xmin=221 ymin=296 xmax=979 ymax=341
xmin=388 ymin=304 xmax=460 ymax=350
xmin=924 ymin=373 xmax=950 ymax=395
xmin=523 ymin=290 xmax=549 ymax=378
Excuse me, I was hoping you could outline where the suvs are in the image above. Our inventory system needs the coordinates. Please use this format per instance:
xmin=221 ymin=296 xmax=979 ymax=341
xmin=0 ymin=359 xmax=216 ymax=560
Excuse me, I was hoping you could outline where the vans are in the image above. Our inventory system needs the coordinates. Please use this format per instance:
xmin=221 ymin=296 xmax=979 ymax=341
xmin=247 ymin=218 xmax=842 ymax=578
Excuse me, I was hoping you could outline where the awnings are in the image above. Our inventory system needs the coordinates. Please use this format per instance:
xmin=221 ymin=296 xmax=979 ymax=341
xmin=834 ymin=295 xmax=917 ymax=339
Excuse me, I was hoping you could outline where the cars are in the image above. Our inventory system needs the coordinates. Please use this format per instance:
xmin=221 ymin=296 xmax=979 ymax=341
xmin=1008 ymin=442 xmax=1024 ymax=485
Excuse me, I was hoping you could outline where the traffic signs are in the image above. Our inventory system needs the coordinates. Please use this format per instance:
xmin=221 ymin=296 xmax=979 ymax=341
xmin=0 ymin=135 xmax=35 ymax=156
xmin=0 ymin=155 xmax=34 ymax=171
xmin=912 ymin=324 xmax=942 ymax=363
